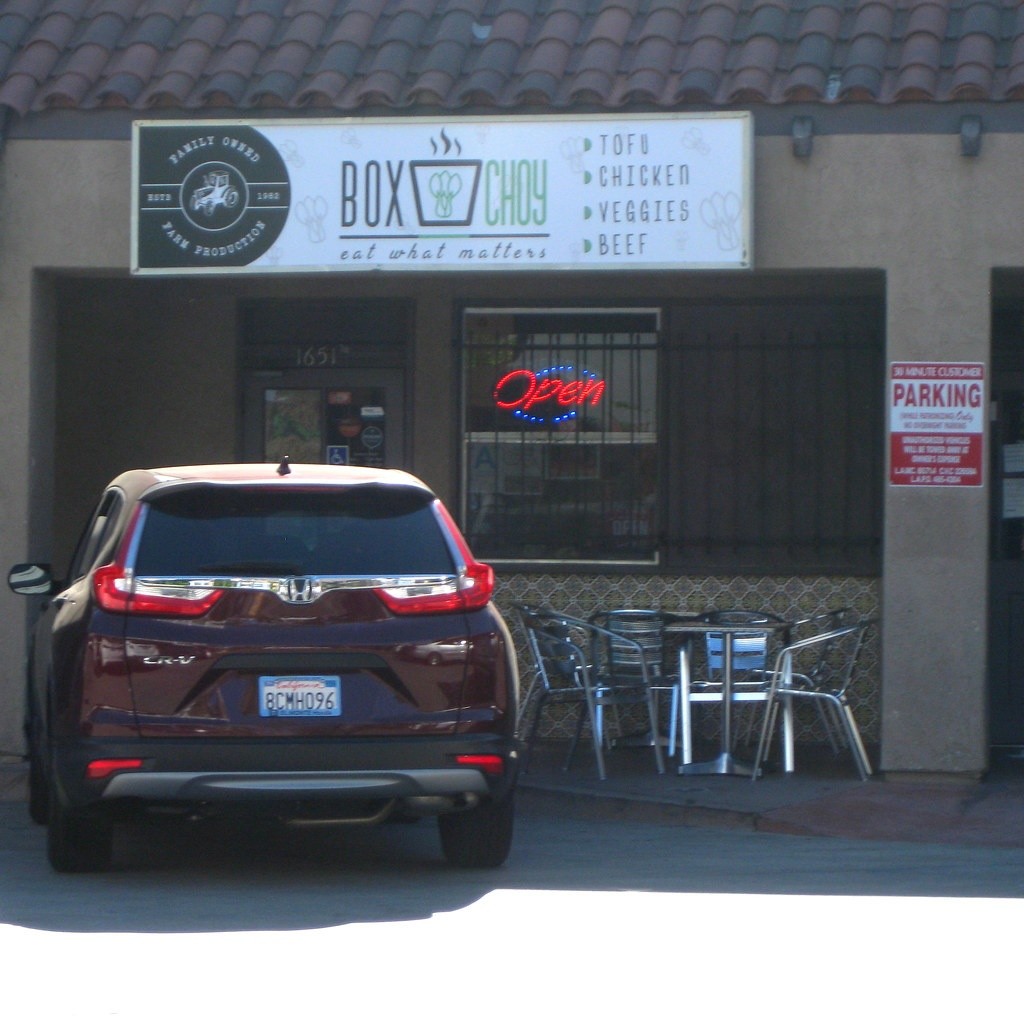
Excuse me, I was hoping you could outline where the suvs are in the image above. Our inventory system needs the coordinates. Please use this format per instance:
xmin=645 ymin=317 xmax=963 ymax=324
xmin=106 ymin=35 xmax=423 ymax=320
xmin=8 ymin=454 xmax=524 ymax=877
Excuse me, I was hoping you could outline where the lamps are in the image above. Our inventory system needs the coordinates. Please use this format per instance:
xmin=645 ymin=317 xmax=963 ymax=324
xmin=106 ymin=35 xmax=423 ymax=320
xmin=792 ymin=116 xmax=814 ymax=155
xmin=961 ymin=114 xmax=982 ymax=155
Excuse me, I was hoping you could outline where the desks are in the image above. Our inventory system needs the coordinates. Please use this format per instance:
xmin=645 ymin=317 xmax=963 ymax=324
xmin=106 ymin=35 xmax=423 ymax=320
xmin=664 ymin=621 xmax=794 ymax=777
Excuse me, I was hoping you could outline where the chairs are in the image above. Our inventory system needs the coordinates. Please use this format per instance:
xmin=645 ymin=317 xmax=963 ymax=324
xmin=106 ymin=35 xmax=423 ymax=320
xmin=510 ymin=603 xmax=873 ymax=786
